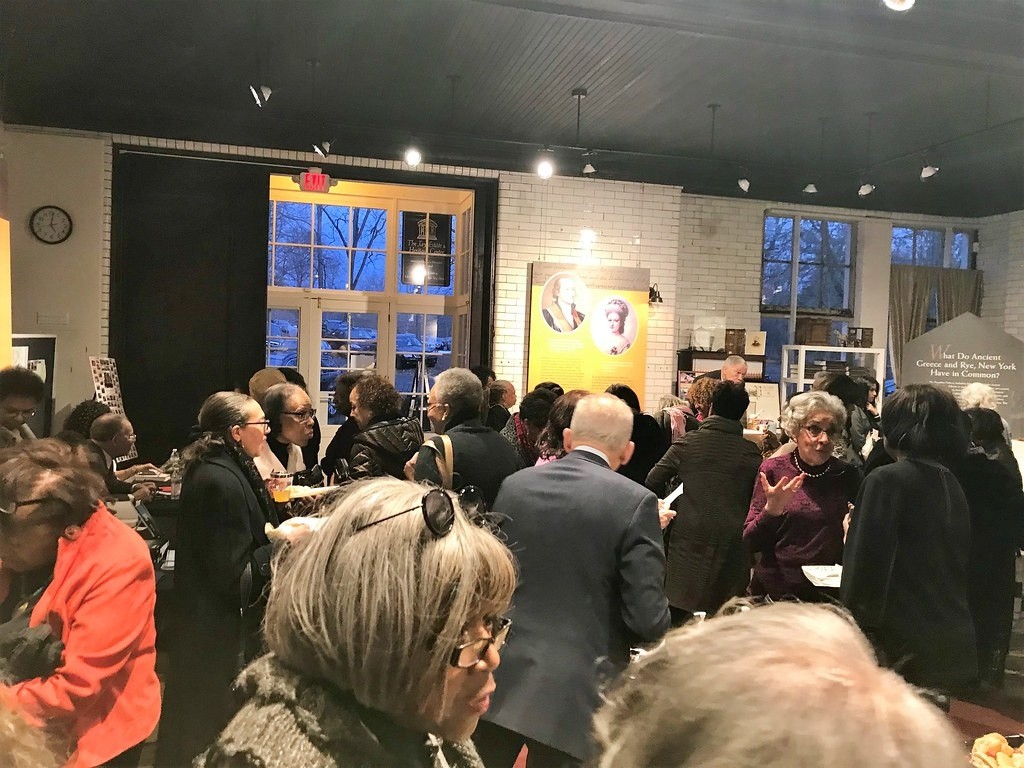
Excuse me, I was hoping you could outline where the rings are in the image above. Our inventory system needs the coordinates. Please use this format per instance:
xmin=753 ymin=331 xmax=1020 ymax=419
xmin=793 ymin=490 xmax=797 ymax=494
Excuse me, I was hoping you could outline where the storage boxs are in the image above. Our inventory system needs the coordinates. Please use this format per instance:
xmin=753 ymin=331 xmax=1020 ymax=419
xmin=795 ymin=317 xmax=832 ymax=346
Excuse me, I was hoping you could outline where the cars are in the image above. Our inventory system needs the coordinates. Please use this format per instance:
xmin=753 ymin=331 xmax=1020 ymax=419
xmin=267 ymin=314 xmax=452 ymax=416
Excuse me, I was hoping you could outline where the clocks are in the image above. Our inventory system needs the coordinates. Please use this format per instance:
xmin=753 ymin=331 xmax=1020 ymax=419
xmin=29 ymin=205 xmax=73 ymax=244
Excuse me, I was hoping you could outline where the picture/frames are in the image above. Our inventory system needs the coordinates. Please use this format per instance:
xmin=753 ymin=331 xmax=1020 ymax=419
xmin=745 ymin=330 xmax=766 ymax=355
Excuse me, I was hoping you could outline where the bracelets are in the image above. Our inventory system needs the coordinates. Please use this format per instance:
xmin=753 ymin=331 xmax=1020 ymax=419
xmin=873 ymin=415 xmax=880 ymax=418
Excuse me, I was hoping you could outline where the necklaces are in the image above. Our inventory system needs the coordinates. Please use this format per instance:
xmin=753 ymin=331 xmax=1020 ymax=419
xmin=793 ymin=449 xmax=832 ymax=477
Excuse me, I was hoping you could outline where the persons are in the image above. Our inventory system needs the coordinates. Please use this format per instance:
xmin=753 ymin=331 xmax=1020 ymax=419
xmin=603 ymin=298 xmax=632 ymax=355
xmin=0 ymin=355 xmax=1024 ymax=768
xmin=542 ymin=278 xmax=586 ymax=332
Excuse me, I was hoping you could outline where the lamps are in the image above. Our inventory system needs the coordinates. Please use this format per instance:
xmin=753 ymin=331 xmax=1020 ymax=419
xmin=534 ymin=145 xmax=555 ymax=179
xmin=737 ymin=165 xmax=752 ymax=192
xmin=802 ymin=170 xmax=822 ymax=193
xmin=855 ymin=172 xmax=875 ymax=195
xmin=250 ymin=38 xmax=272 ymax=117
xmin=919 ymin=149 xmax=940 ymax=178
xmin=312 ymin=136 xmax=338 ymax=158
xmin=581 ymin=151 xmax=597 ymax=173
xmin=649 ymin=283 xmax=663 ymax=303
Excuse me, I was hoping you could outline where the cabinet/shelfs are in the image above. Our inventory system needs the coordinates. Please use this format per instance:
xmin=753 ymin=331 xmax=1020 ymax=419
xmin=779 ymin=345 xmax=885 ymax=418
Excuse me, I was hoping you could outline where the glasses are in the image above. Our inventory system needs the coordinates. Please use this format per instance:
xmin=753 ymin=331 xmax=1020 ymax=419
xmin=429 ymin=615 xmax=513 ymax=668
xmin=281 ymin=409 xmax=317 ymax=420
xmin=230 ymin=419 xmax=270 ymax=431
xmin=118 ymin=433 xmax=137 ymax=440
xmin=0 ymin=404 xmax=37 ymax=418
xmin=356 ymin=484 xmax=488 ymax=537
xmin=800 ymin=425 xmax=840 ymax=438
xmin=425 ymin=397 xmax=451 ymax=412
xmin=0 ymin=495 xmax=54 ymax=514
